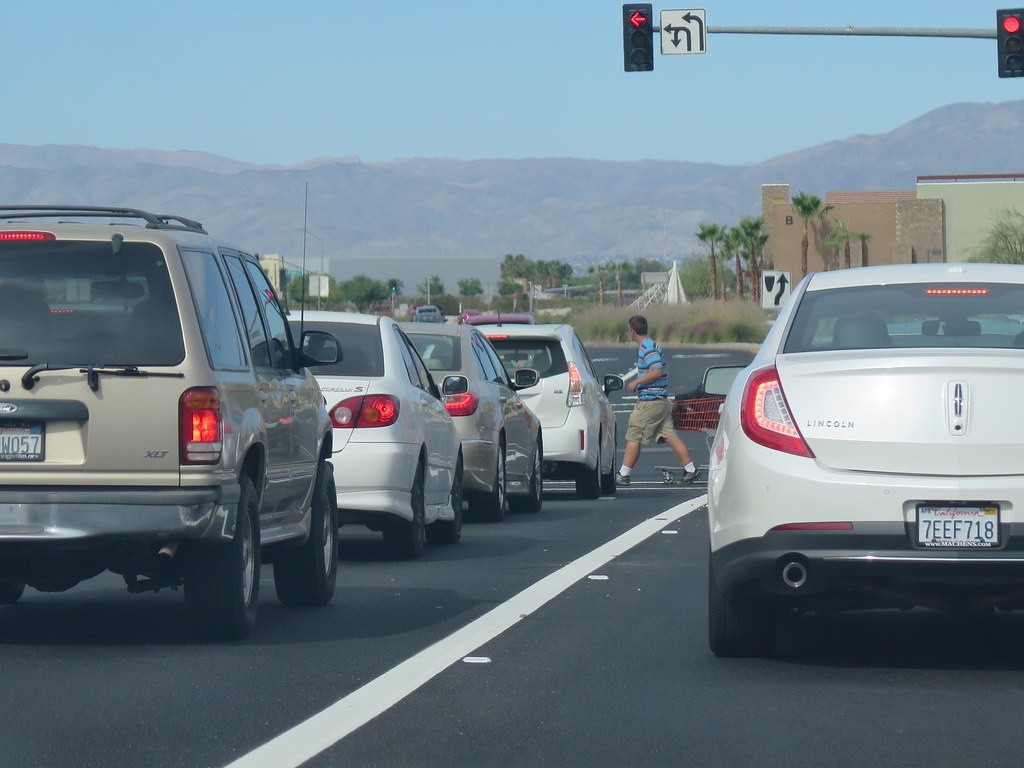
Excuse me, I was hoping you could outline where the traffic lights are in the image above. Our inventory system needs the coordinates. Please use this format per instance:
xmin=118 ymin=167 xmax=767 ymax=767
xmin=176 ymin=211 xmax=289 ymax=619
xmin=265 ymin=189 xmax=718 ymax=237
xmin=622 ymin=3 xmax=654 ymax=72
xmin=995 ymin=7 xmax=1024 ymax=79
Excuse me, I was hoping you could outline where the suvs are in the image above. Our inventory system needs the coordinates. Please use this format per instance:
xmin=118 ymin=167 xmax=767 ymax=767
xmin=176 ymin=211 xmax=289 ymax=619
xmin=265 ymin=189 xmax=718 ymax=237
xmin=1 ymin=201 xmax=342 ymax=647
xmin=421 ymin=321 xmax=626 ymax=502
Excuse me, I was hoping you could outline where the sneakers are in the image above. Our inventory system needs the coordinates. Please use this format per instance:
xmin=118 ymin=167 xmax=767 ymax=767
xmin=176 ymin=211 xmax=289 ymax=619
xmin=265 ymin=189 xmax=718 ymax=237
xmin=680 ymin=470 xmax=702 ymax=485
xmin=615 ymin=472 xmax=631 ymax=487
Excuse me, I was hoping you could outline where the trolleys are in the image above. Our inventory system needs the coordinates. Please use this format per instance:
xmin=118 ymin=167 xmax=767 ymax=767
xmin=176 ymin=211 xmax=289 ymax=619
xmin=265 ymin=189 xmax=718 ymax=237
xmin=653 ymin=394 xmax=725 ymax=486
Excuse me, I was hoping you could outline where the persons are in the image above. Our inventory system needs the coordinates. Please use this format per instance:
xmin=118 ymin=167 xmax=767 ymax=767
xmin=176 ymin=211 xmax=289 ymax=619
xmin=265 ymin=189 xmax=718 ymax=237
xmin=614 ymin=312 xmax=702 ymax=487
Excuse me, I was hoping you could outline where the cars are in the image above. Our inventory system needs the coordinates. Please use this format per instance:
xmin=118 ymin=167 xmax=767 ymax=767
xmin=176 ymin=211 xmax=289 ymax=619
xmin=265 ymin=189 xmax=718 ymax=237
xmin=282 ymin=289 xmax=471 ymax=564
xmin=414 ymin=305 xmax=445 ymax=324
xmin=372 ymin=305 xmax=395 ymax=318
xmin=405 ymin=304 xmax=445 ymax=319
xmin=459 ymin=313 xmax=541 ymax=377
xmin=696 ymin=262 xmax=1024 ymax=664
xmin=393 ymin=320 xmax=544 ymax=525
